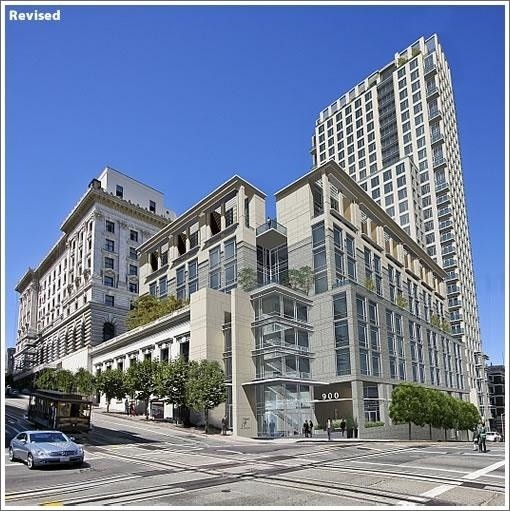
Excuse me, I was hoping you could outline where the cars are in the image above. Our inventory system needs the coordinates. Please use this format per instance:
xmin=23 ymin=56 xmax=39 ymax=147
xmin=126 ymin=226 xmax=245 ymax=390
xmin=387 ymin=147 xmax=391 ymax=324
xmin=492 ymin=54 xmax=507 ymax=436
xmin=485 ymin=432 xmax=502 ymax=441
xmin=8 ymin=429 xmax=84 ymax=468
